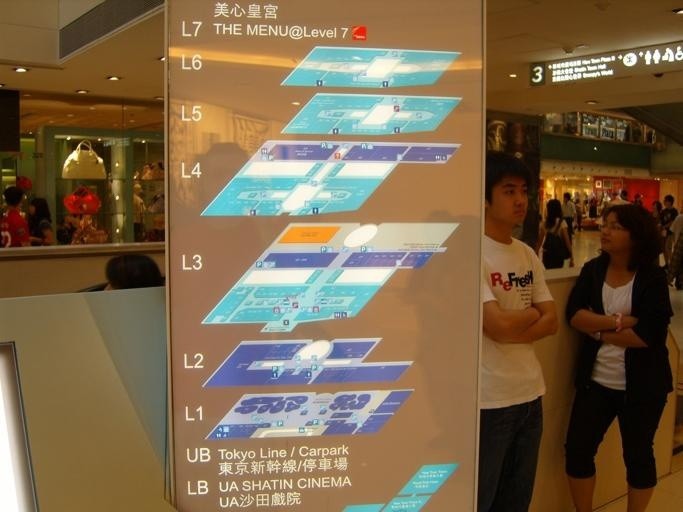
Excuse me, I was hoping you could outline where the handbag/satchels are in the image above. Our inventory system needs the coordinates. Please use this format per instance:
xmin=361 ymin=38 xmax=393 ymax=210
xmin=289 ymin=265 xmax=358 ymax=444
xmin=147 ymin=193 xmax=164 ymax=212
xmin=64 ymin=186 xmax=102 ymax=214
xmin=72 ymin=215 xmax=96 ymax=244
xmin=142 ymin=161 xmax=165 ymax=180
xmin=543 ymin=233 xmax=570 ymax=259
xmin=62 ymin=138 xmax=108 ymax=180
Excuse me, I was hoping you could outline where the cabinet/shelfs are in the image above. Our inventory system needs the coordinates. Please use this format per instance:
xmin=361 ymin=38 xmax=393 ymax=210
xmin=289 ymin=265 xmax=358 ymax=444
xmin=46 ymin=128 xmax=163 ymax=244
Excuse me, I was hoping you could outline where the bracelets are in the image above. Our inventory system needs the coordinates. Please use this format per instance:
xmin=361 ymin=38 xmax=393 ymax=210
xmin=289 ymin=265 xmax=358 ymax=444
xmin=593 ymin=330 xmax=600 ymax=341
xmin=615 ymin=312 xmax=623 ymax=332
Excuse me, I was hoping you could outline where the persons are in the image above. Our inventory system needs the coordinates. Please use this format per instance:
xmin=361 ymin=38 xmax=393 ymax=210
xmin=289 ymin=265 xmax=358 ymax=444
xmin=478 ymin=149 xmax=559 ymax=512
xmin=1 ymin=183 xmax=163 ymax=291
xmin=534 ymin=191 xmax=683 ymax=291
xmin=564 ymin=204 xmax=674 ymax=512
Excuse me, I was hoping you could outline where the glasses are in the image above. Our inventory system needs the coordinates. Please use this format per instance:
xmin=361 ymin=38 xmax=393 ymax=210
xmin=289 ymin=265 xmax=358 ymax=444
xmin=599 ymin=222 xmax=625 ymax=231
